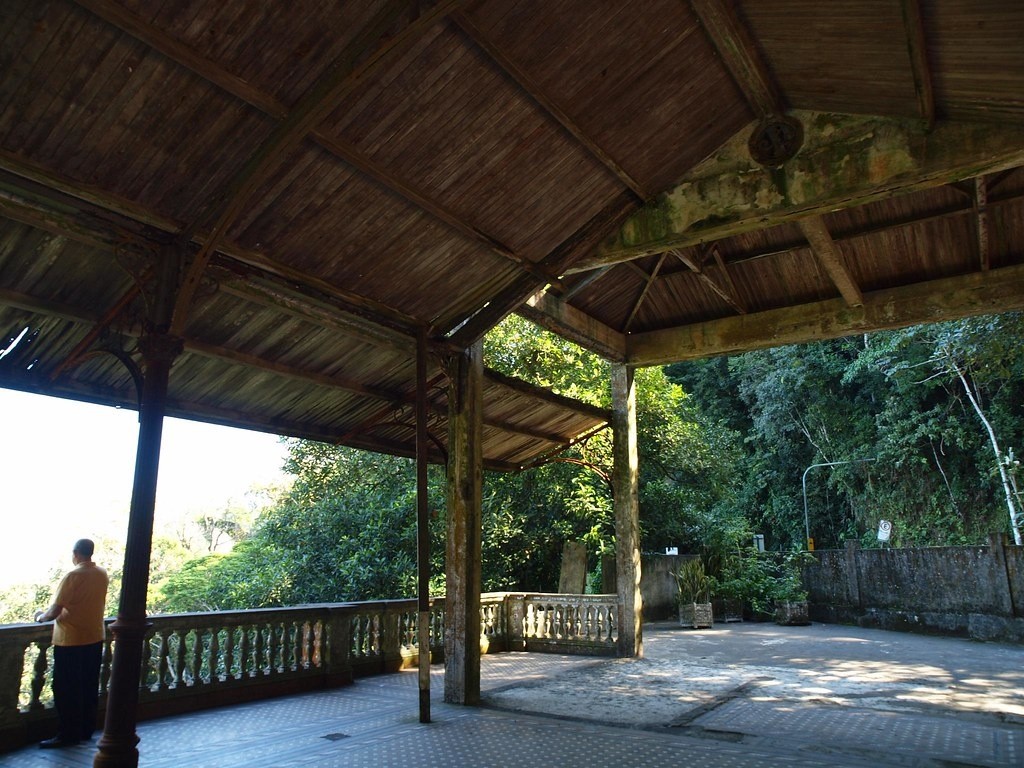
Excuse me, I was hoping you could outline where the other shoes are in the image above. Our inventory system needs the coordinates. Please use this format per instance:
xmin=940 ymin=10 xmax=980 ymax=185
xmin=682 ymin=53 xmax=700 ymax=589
xmin=74 ymin=733 xmax=91 ymax=741
xmin=40 ymin=736 xmax=73 ymax=747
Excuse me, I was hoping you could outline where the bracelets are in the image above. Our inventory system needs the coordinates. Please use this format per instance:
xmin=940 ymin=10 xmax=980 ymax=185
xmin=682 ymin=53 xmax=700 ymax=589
xmin=37 ymin=615 xmax=43 ymax=623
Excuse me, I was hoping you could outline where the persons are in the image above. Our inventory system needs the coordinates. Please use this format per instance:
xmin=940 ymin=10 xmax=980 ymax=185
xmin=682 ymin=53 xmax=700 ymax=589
xmin=35 ymin=539 xmax=109 ymax=747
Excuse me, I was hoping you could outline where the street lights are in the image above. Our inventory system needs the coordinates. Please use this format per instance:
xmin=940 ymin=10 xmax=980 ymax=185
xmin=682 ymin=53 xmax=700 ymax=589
xmin=803 ymin=458 xmax=875 ymax=550
